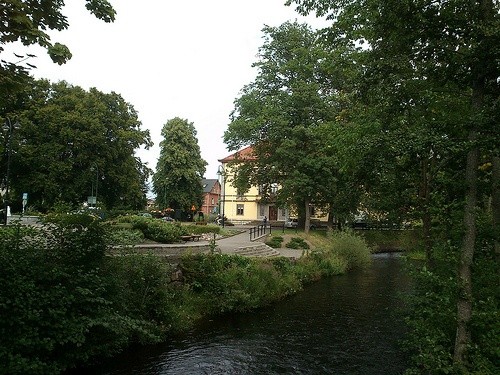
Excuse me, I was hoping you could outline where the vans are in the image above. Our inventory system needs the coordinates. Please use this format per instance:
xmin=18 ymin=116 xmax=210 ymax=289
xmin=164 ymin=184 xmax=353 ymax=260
xmin=287 ymin=216 xmax=297 ymax=228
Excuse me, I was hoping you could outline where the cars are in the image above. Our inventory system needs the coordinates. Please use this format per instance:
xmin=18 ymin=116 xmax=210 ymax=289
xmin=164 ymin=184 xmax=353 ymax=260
xmin=162 ymin=217 xmax=173 ymax=222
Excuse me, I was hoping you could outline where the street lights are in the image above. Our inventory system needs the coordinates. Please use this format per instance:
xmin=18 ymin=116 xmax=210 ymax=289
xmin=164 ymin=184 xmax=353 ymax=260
xmin=217 ymin=165 xmax=226 ymax=228
xmin=90 ymin=161 xmax=98 ymax=209
